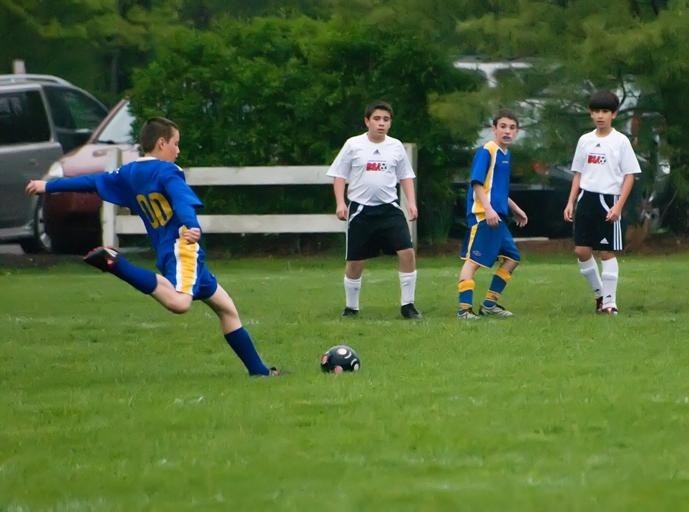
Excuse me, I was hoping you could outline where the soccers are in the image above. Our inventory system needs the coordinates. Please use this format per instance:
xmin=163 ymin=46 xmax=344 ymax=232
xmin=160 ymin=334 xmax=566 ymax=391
xmin=320 ymin=345 xmax=360 ymax=374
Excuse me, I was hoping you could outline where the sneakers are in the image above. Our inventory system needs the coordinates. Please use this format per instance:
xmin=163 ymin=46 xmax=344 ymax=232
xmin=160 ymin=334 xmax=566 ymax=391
xmin=596 ymin=296 xmax=618 ymax=315
xmin=342 ymin=307 xmax=359 ymax=318
xmin=400 ymin=304 xmax=422 ymax=319
xmin=83 ymin=245 xmax=118 ymax=271
xmin=261 ymin=367 xmax=281 ymax=379
xmin=458 ymin=304 xmax=513 ymax=320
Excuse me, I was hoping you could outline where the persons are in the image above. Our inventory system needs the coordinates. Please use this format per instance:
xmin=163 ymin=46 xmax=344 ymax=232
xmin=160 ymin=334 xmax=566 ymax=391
xmin=26 ymin=114 xmax=285 ymax=378
xmin=321 ymin=98 xmax=423 ymax=320
xmin=456 ymin=106 xmax=530 ymax=317
xmin=561 ymin=89 xmax=647 ymax=313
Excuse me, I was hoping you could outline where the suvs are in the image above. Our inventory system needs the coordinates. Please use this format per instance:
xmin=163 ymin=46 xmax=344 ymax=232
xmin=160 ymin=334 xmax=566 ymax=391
xmin=1 ymin=71 xmax=171 ymax=256
xmin=443 ymin=55 xmax=646 ymax=182
